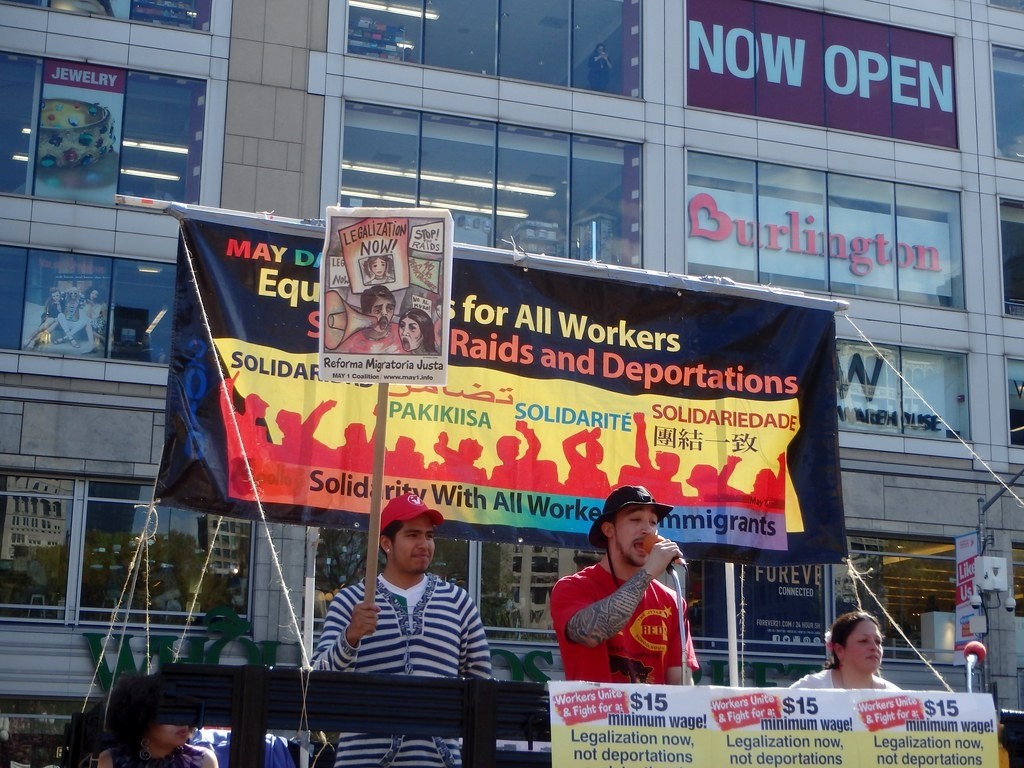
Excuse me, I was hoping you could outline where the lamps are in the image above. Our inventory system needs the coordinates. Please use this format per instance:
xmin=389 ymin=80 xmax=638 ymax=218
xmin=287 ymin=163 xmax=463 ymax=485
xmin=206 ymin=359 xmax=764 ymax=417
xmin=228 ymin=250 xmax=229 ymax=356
xmin=349 ymin=0 xmax=387 ymax=15
xmin=386 ymin=6 xmax=440 ymax=20
xmin=342 ymin=160 xmax=557 ymax=219
xmin=12 ymin=127 xmax=189 ymax=180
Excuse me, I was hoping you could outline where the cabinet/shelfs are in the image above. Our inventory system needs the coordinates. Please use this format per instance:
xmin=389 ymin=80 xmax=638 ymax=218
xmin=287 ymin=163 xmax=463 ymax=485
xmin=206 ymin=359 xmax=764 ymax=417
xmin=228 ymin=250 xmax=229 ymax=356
xmin=348 ymin=20 xmax=405 ymax=61
xmin=511 ymin=221 xmax=558 ymax=257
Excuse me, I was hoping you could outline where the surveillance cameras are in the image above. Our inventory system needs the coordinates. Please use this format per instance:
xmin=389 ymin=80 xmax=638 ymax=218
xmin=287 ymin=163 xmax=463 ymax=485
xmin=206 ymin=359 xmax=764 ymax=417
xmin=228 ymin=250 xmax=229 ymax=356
xmin=971 ymin=595 xmax=982 ymax=609
xmin=1004 ymin=597 xmax=1016 ymax=612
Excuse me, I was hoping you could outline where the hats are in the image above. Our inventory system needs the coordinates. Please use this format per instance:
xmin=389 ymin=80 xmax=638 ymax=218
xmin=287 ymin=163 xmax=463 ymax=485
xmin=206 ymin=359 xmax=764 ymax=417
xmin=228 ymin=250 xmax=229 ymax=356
xmin=380 ymin=493 xmax=444 ymax=532
xmin=588 ymin=485 xmax=674 ymax=548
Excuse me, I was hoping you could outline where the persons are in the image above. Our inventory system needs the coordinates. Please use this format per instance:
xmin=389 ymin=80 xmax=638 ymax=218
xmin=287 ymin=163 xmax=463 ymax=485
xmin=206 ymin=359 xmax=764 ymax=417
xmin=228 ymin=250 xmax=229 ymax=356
xmin=588 ymin=44 xmax=613 ymax=92
xmin=24 ymin=285 xmax=109 ymax=354
xmin=789 ymin=611 xmax=903 ymax=691
xmin=15 ymin=553 xmax=52 ymax=612
xmin=311 ymin=493 xmax=492 ymax=768
xmin=96 ymin=673 xmax=219 ymax=768
xmin=549 ymin=485 xmax=700 ymax=686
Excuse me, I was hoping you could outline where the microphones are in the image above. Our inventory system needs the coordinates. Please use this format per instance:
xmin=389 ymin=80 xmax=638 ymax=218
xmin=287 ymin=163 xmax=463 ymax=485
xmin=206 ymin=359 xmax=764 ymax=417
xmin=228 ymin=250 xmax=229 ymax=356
xmin=963 ymin=641 xmax=986 ymax=672
xmin=643 ymin=534 xmax=686 ymax=567
